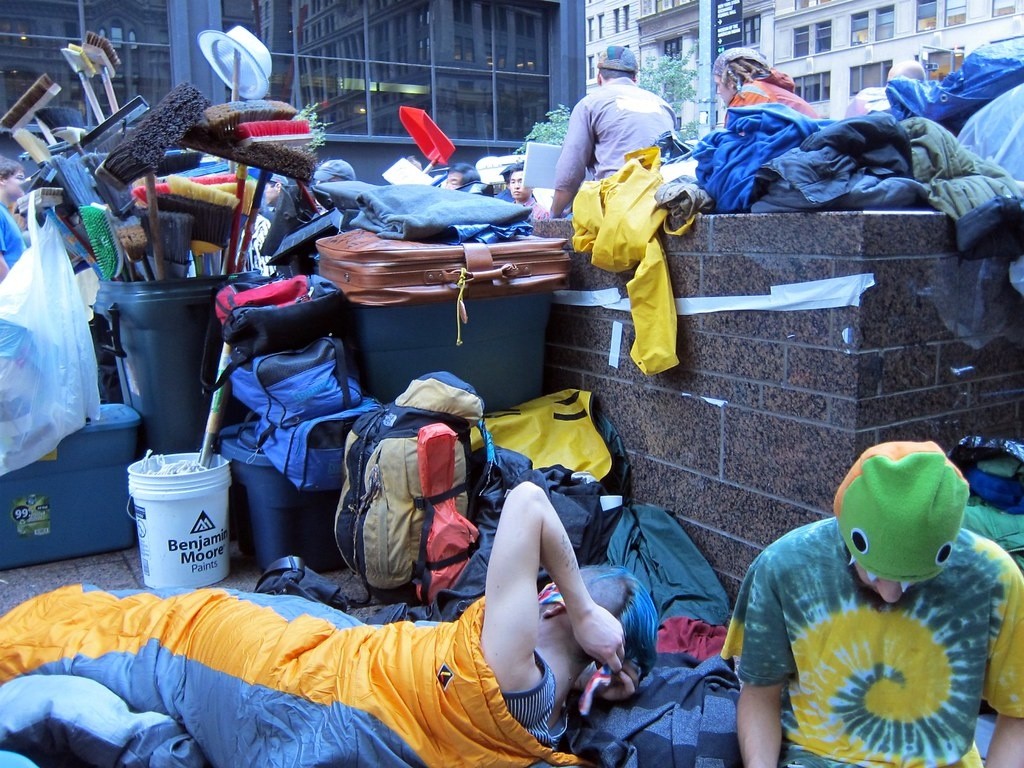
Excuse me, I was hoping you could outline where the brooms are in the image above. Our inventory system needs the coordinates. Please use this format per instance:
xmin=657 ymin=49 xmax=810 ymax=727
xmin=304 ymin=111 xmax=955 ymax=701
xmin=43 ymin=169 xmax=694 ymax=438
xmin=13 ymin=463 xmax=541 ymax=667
xmin=0 ymin=31 xmax=320 ymax=281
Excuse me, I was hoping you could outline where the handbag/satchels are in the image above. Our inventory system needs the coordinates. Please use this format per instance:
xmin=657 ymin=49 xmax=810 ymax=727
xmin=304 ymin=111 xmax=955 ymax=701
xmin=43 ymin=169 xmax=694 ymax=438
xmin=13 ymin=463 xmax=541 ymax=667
xmin=310 ymin=225 xmax=571 ymax=306
xmin=221 ymin=336 xmax=382 ymax=492
xmin=0 ymin=189 xmax=103 ymax=477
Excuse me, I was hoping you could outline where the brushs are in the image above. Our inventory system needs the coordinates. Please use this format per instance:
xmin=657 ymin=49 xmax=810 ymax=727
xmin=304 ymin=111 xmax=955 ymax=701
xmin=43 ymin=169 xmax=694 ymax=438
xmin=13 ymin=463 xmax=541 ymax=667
xmin=178 ymin=99 xmax=318 ymax=275
xmin=79 ymin=201 xmax=125 ymax=282
xmin=58 ymin=30 xmax=122 ymax=126
xmin=94 ymin=81 xmax=212 ymax=192
xmin=118 ymin=225 xmax=155 ymax=284
xmin=15 ymin=164 xmax=104 ymax=285
xmin=0 ymin=73 xmax=64 ymax=146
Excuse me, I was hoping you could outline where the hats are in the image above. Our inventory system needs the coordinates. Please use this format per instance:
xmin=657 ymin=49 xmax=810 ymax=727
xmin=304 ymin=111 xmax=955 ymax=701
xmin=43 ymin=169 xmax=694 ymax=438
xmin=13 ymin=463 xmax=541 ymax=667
xmin=314 ymin=160 xmax=356 ymax=182
xmin=197 ymin=25 xmax=272 ymax=99
xmin=831 ymin=440 xmax=972 ymax=593
xmin=599 ymin=44 xmax=638 ymax=70
xmin=713 ymin=49 xmax=769 ymax=79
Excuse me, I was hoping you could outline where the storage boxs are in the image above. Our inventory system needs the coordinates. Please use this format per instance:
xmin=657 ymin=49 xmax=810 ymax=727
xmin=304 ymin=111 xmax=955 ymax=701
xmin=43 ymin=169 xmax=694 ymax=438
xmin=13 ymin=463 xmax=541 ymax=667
xmin=0 ymin=403 xmax=142 ymax=570
xmin=222 ymin=439 xmax=348 ymax=572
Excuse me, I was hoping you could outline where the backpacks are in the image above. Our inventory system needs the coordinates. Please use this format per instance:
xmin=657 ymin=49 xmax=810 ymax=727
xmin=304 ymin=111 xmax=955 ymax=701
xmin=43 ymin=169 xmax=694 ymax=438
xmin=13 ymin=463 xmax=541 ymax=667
xmin=200 ymin=272 xmax=348 ymax=391
xmin=332 ymin=404 xmax=486 ymax=609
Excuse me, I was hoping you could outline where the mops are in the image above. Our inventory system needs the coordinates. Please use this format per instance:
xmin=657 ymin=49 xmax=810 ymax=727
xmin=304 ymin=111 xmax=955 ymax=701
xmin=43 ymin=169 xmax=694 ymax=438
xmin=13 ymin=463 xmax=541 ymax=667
xmin=138 ymin=341 xmax=235 ymax=476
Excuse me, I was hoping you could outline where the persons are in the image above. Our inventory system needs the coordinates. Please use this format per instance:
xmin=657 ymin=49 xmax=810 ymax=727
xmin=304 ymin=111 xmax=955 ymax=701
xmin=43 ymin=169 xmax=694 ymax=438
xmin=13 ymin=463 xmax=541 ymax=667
xmin=316 ymin=159 xmax=356 ymax=182
xmin=509 ymin=164 xmax=549 ymax=221
xmin=264 ymin=174 xmax=288 ymax=206
xmin=0 ymin=156 xmax=26 ymax=283
xmin=446 ymin=163 xmax=481 ymax=190
xmin=481 ymin=480 xmax=661 ymax=763
xmin=722 ymin=441 xmax=1024 ymax=768
xmin=550 ymin=46 xmax=678 ymax=220
xmin=713 ymin=46 xmax=821 ymax=129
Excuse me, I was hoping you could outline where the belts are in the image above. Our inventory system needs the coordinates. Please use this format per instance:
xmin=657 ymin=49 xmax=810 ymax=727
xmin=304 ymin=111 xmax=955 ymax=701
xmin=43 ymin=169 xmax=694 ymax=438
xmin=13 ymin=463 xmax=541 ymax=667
xmin=253 ymin=557 xmax=306 ymax=592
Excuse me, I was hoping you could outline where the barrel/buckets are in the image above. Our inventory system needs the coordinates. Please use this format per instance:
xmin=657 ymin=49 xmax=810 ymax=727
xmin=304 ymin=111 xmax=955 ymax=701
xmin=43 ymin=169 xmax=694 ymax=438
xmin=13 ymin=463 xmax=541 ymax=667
xmin=92 ymin=276 xmax=238 ymax=453
xmin=126 ymin=453 xmax=233 ymax=590
